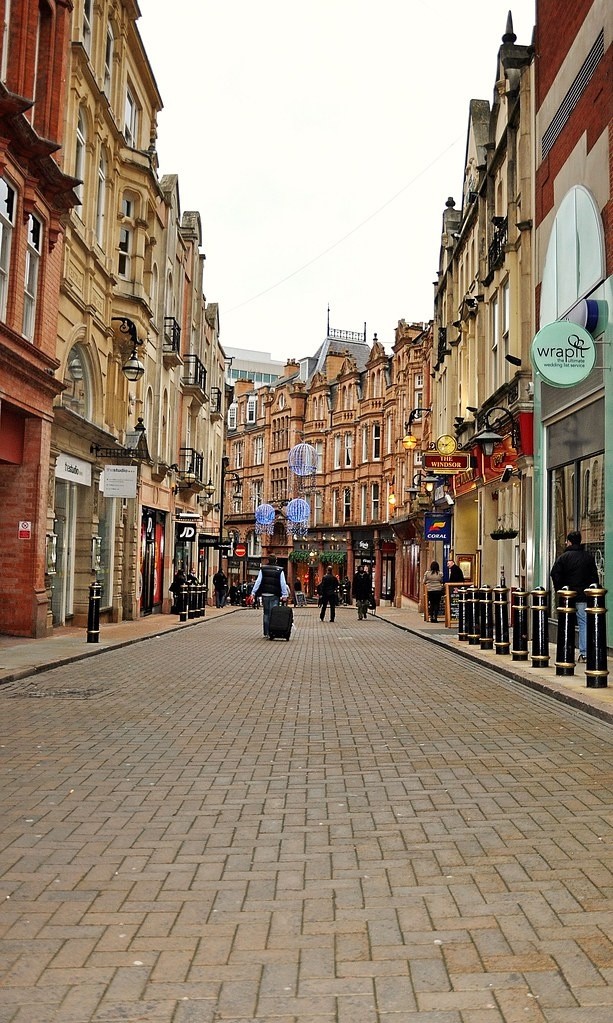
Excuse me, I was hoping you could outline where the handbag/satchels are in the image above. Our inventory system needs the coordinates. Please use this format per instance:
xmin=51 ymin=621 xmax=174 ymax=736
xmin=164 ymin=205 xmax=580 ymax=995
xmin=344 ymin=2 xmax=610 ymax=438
xmin=318 ymin=595 xmax=323 ymax=608
xmin=169 ymin=582 xmax=177 ymax=593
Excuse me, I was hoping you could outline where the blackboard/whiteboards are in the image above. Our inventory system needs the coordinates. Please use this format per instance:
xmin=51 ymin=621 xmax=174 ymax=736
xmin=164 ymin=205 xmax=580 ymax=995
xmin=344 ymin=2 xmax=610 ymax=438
xmin=444 ymin=583 xmax=474 ymax=624
xmin=424 ymin=584 xmax=446 ymax=618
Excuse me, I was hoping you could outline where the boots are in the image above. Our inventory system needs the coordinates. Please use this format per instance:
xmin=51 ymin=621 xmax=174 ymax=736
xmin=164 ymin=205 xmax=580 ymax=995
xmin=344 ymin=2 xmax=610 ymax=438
xmin=254 ymin=606 xmax=256 ymax=609
xmin=257 ymin=605 xmax=259 ymax=609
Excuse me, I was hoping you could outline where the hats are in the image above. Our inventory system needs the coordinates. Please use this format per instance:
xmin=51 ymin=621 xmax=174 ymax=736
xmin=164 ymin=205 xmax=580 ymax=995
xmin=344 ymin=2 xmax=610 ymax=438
xmin=568 ymin=531 xmax=582 ymax=544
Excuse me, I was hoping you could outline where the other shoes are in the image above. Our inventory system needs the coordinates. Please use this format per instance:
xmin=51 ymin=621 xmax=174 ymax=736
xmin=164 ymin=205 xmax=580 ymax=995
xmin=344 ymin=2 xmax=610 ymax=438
xmin=220 ymin=604 xmax=223 ymax=608
xmin=578 ymin=655 xmax=587 ymax=663
xmin=431 ymin=619 xmax=439 ymax=623
xmin=330 ymin=620 xmax=334 ymax=622
xmin=358 ymin=618 xmax=362 ymax=620
xmin=362 ymin=618 xmax=366 ymax=620
xmin=263 ymin=634 xmax=268 ymax=638
xmin=217 ymin=607 xmax=220 ymax=608
xmin=321 ymin=619 xmax=324 ymax=621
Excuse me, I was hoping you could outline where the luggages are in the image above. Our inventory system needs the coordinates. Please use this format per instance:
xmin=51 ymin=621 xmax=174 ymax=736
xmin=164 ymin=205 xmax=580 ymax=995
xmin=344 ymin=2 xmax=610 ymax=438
xmin=267 ymin=600 xmax=293 ymax=641
xmin=246 ymin=596 xmax=254 ymax=607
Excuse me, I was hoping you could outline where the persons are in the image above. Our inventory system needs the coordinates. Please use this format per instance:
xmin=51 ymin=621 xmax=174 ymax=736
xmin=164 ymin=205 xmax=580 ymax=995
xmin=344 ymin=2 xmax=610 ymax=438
xmin=550 ymin=530 xmax=599 ymax=663
xmin=422 ymin=560 xmax=443 ymax=623
xmin=445 ymin=560 xmax=465 ymax=582
xmin=223 ymin=578 xmax=263 ymax=609
xmin=250 ymin=553 xmax=287 ymax=639
xmin=174 ymin=567 xmax=198 ymax=615
xmin=317 ymin=574 xmax=351 ymax=608
xmin=213 ymin=568 xmax=228 ymax=608
xmin=319 ymin=566 xmax=338 ymax=622
xmin=352 ymin=565 xmax=372 ymax=620
xmin=282 ymin=577 xmax=303 ymax=608
